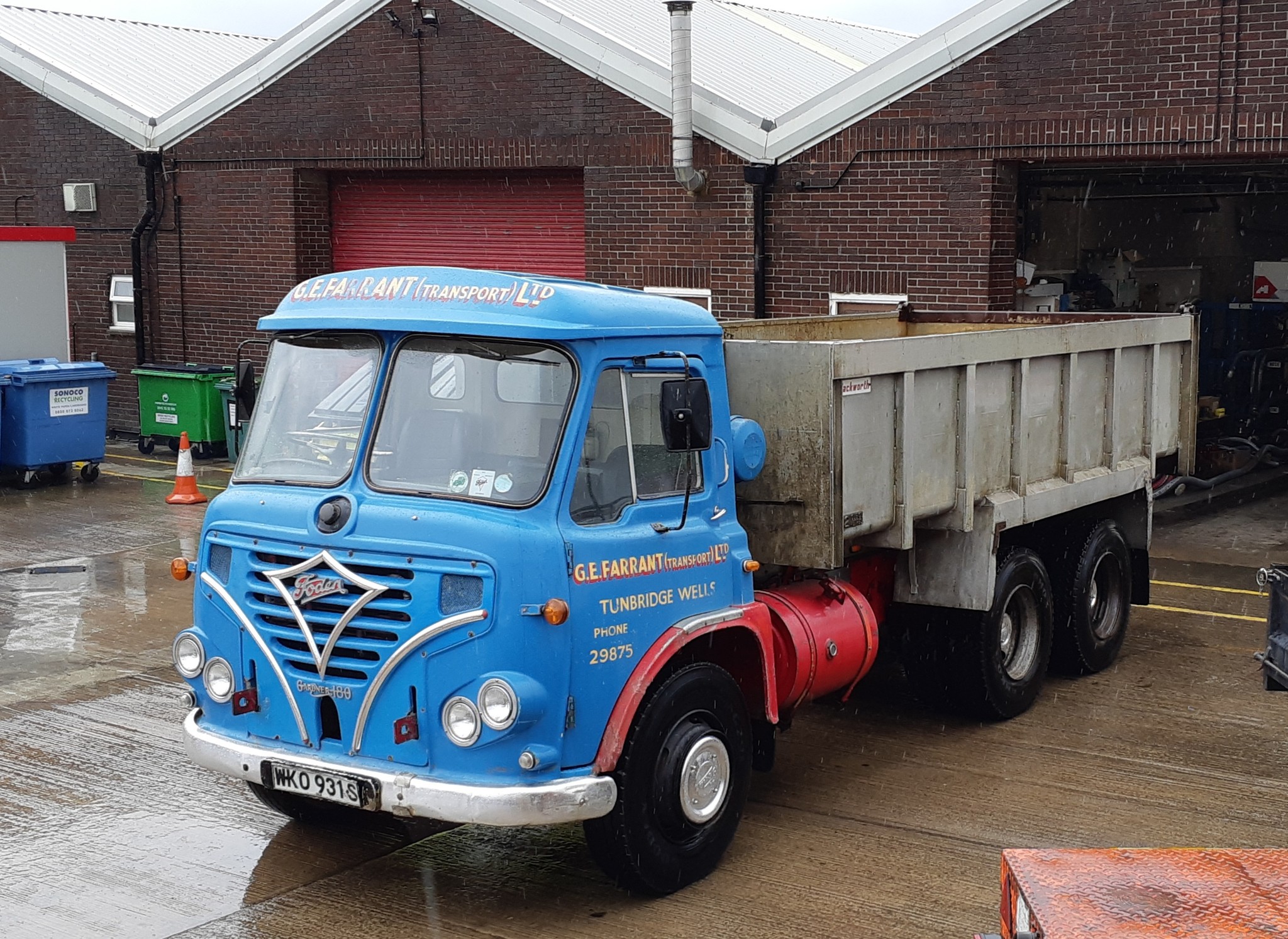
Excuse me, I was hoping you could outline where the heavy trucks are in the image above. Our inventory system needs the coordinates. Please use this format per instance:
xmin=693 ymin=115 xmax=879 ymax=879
xmin=171 ymin=265 xmax=1201 ymax=901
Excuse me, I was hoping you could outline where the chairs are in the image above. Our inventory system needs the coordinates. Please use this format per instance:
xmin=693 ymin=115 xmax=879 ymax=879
xmin=397 ymin=406 xmax=475 ymax=488
xmin=592 ymin=443 xmax=692 ymax=518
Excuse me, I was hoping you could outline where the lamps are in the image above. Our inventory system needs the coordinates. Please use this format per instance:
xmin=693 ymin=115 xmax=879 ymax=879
xmin=385 ymin=9 xmax=419 ymax=36
xmin=422 ymin=7 xmax=441 ymax=37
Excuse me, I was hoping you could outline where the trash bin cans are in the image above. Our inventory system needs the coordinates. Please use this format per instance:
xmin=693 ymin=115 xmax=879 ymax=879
xmin=214 ymin=377 xmax=264 ymax=463
xmin=0 ymin=358 xmax=117 ymax=489
xmin=131 ymin=363 xmax=235 ymax=461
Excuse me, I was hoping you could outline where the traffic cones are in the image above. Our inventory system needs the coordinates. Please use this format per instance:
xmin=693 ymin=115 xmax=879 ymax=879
xmin=165 ymin=432 xmax=209 ymax=505
